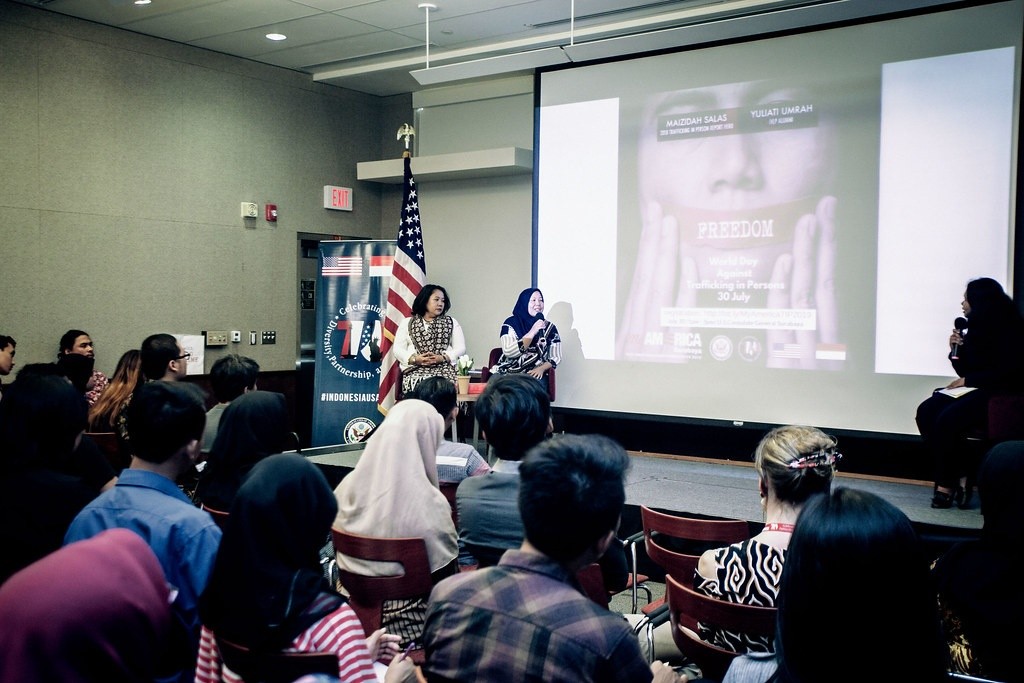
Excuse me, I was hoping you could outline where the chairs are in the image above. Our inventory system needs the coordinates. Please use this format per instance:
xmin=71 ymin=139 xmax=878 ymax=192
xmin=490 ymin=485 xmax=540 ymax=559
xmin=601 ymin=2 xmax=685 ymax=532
xmin=484 ymin=347 xmax=556 ymax=461
xmin=932 ymin=379 xmax=1024 ymax=495
xmin=77 ymin=430 xmax=784 ymax=683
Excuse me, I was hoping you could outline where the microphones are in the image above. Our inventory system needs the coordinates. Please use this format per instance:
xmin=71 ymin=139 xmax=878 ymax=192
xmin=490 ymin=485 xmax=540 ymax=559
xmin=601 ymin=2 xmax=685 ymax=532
xmin=536 ymin=312 xmax=545 ymax=342
xmin=952 ymin=317 xmax=966 ymax=359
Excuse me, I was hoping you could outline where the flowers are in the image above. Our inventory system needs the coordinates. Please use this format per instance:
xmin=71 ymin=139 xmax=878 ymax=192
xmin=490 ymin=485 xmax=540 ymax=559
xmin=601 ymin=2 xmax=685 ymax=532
xmin=457 ymin=354 xmax=474 ymax=376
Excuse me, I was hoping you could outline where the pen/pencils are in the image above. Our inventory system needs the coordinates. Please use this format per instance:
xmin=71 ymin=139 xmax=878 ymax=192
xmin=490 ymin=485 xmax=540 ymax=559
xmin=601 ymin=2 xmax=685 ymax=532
xmin=399 ymin=642 xmax=415 ymax=662
xmin=662 ymin=662 xmax=669 ymax=666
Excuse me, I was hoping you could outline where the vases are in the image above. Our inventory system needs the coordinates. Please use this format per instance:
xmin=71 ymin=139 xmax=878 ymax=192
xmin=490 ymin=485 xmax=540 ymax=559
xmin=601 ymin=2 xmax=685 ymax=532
xmin=457 ymin=375 xmax=471 ymax=395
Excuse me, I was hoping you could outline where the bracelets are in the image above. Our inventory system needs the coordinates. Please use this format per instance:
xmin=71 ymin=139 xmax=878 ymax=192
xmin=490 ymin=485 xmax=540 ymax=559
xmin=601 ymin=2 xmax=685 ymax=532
xmin=440 ymin=353 xmax=448 ymax=366
xmin=412 ymin=356 xmax=416 ymax=366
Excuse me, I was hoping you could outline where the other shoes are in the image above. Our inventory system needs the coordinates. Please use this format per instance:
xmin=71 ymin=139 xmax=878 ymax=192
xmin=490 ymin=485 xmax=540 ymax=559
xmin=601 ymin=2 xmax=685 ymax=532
xmin=930 ymin=491 xmax=953 ymax=509
xmin=953 ymin=486 xmax=964 ymax=503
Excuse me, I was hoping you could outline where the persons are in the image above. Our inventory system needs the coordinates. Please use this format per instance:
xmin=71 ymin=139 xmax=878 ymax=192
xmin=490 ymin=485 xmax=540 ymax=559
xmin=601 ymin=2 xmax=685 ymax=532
xmin=0 ymin=330 xmax=1022 ymax=682
xmin=915 ymin=278 xmax=1024 ymax=509
xmin=392 ymin=284 xmax=466 ymax=400
xmin=483 ymin=288 xmax=562 ymax=433
xmin=616 ymin=68 xmax=847 ymax=367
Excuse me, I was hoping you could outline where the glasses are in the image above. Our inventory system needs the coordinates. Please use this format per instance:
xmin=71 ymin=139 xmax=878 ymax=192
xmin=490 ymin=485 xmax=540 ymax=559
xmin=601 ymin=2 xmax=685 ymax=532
xmin=168 ymin=583 xmax=179 ymax=603
xmin=176 ymin=351 xmax=191 ymax=360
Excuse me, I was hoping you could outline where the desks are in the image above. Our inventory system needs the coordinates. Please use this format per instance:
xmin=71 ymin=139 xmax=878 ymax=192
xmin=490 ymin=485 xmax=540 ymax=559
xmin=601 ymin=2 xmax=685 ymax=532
xmin=452 ymin=395 xmax=487 ymax=447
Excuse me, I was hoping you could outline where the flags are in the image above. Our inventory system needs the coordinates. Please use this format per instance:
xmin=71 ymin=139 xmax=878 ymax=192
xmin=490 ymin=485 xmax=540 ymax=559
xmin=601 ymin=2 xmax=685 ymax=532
xmin=378 ymin=156 xmax=428 ymax=418
